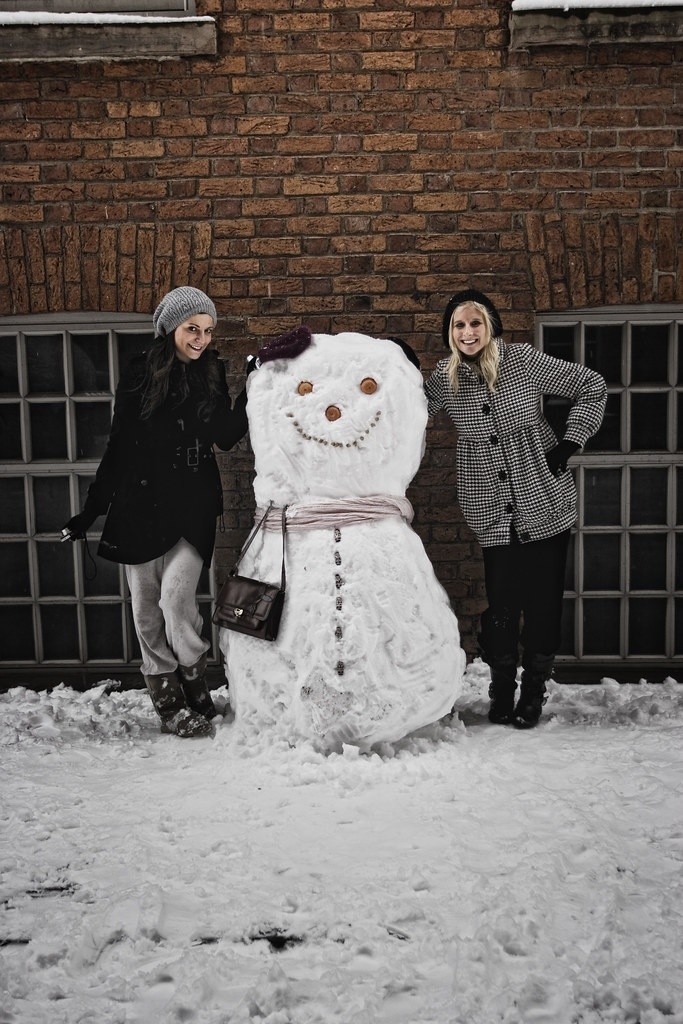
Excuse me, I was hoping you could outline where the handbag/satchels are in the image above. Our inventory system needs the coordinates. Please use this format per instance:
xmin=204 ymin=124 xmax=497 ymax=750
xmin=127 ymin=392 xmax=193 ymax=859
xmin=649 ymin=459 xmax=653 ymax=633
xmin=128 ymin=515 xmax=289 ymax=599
xmin=212 ymin=575 xmax=286 ymax=641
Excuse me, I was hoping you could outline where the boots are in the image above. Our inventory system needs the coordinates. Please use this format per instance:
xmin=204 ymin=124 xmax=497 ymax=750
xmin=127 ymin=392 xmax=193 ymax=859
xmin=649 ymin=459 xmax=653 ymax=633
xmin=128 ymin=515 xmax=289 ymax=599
xmin=143 ymin=655 xmax=217 ymax=737
xmin=483 ymin=651 xmax=555 ymax=729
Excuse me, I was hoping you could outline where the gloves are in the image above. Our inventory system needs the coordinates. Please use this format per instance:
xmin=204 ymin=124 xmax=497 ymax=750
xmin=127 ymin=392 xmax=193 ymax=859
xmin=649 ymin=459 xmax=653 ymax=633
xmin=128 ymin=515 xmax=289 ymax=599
xmin=386 ymin=337 xmax=420 ymax=371
xmin=246 ymin=356 xmax=258 ymax=378
xmin=545 ymin=440 xmax=581 ymax=477
xmin=61 ymin=511 xmax=98 ymax=541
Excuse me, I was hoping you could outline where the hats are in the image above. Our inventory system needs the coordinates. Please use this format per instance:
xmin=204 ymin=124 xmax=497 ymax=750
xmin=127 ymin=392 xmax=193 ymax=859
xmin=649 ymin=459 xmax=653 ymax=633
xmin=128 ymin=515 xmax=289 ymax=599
xmin=152 ymin=286 xmax=217 ymax=336
xmin=442 ymin=289 xmax=503 ymax=348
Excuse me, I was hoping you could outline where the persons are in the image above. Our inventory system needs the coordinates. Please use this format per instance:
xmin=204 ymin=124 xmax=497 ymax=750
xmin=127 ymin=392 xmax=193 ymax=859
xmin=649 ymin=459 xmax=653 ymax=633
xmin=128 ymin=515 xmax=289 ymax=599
xmin=387 ymin=292 xmax=607 ymax=729
xmin=60 ymin=287 xmax=312 ymax=738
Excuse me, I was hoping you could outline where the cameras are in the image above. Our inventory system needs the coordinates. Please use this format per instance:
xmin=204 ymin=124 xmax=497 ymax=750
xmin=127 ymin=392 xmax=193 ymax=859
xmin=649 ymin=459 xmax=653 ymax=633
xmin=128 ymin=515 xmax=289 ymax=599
xmin=60 ymin=527 xmax=73 ymax=544
